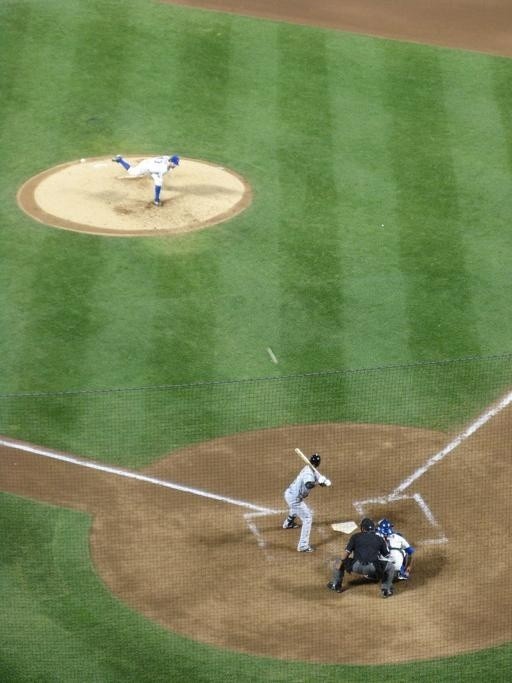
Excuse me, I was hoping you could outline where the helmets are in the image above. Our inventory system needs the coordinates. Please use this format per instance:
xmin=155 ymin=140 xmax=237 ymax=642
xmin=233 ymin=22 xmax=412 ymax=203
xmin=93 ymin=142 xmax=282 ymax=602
xmin=376 ymin=518 xmax=394 ymax=535
xmin=309 ymin=453 xmax=322 ymax=467
xmin=171 ymin=155 xmax=179 ymax=165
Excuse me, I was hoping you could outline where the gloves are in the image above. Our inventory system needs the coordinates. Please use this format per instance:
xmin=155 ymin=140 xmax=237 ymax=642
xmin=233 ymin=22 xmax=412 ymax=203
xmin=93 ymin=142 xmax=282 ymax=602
xmin=318 ymin=475 xmax=331 ymax=487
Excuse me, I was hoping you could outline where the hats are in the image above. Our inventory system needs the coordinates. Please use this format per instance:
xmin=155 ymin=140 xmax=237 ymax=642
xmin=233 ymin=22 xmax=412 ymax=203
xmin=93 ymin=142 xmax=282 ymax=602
xmin=362 ymin=518 xmax=376 ymax=533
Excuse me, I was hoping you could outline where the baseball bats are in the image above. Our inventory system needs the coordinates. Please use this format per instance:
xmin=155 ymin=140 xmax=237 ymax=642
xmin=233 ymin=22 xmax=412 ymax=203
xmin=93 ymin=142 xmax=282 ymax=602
xmin=294 ymin=448 xmax=322 ymax=476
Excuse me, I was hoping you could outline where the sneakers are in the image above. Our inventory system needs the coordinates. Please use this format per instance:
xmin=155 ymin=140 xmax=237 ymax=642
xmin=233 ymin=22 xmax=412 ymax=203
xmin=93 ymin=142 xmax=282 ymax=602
xmin=282 ymin=524 xmax=300 ymax=529
xmin=303 ymin=546 xmax=314 ymax=553
xmin=111 ymin=155 xmax=122 ymax=161
xmin=153 ymin=199 xmax=161 ymax=205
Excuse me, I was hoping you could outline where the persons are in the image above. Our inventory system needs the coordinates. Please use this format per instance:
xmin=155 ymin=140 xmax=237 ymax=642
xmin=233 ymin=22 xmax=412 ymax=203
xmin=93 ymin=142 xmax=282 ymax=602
xmin=112 ymin=155 xmax=180 ymax=206
xmin=375 ymin=519 xmax=415 ymax=580
xmin=283 ymin=454 xmax=331 ymax=552
xmin=326 ymin=518 xmax=395 ymax=598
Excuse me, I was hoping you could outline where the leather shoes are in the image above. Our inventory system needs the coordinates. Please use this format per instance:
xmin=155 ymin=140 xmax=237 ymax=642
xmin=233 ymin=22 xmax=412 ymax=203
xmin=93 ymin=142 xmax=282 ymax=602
xmin=327 ymin=581 xmax=342 ymax=593
xmin=383 ymin=590 xmax=393 ymax=598
